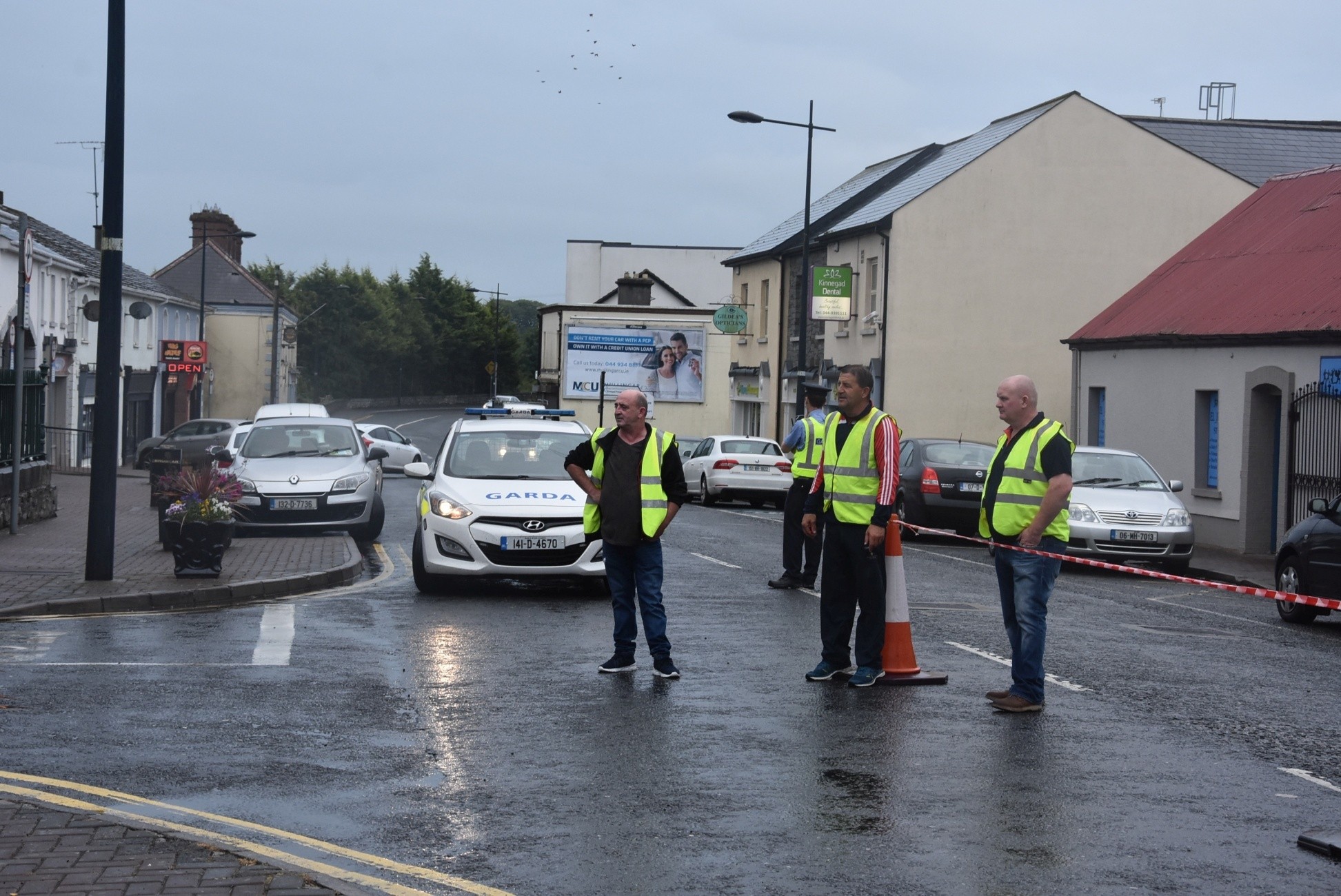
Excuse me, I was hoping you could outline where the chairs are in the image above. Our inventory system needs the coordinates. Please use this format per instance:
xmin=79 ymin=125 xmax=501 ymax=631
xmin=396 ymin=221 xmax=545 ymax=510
xmin=257 ymin=428 xmax=291 ymax=455
xmin=724 ymin=443 xmax=737 ymax=453
xmin=960 ymin=452 xmax=978 ymax=466
xmin=1081 ymin=465 xmax=1101 ymax=480
xmin=926 ymin=447 xmax=940 ymax=462
xmin=501 ymin=451 xmax=526 ymax=472
xmin=535 ymin=440 xmax=570 ymax=474
xmin=464 ymin=441 xmax=494 ymax=473
xmin=324 ymin=428 xmax=346 ymax=451
xmin=748 ymin=444 xmax=761 ymax=453
xmin=299 ymin=438 xmax=321 ymax=452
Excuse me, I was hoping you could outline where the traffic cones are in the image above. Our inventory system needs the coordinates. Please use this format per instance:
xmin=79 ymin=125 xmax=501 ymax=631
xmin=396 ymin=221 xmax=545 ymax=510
xmin=877 ymin=513 xmax=949 ymax=687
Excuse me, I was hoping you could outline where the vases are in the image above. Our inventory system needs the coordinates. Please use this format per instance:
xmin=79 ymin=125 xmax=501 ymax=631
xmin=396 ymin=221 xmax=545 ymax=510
xmin=158 ymin=516 xmax=237 ymax=576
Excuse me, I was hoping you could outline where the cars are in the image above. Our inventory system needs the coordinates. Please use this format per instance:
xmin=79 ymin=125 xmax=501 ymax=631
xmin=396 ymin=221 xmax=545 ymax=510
xmin=483 ymin=395 xmax=521 ymax=409
xmin=403 ymin=402 xmax=611 ymax=601
xmin=134 ymin=403 xmax=389 ymax=542
xmin=356 ymin=423 xmax=433 ymax=472
xmin=1274 ymin=491 xmax=1341 ymax=625
xmin=676 ymin=435 xmax=794 ymax=511
xmin=896 ymin=437 xmax=998 ymax=541
xmin=1059 ymin=445 xmax=1196 ymax=574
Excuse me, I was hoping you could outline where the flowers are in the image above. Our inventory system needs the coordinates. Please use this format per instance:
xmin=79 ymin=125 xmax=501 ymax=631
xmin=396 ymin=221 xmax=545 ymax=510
xmin=162 ymin=463 xmax=249 ymax=529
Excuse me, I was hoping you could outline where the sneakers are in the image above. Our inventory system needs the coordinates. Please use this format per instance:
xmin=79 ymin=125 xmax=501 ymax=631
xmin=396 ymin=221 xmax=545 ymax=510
xmin=848 ymin=666 xmax=885 ymax=687
xmin=806 ymin=660 xmax=852 ymax=680
xmin=986 ymin=690 xmax=1010 ymax=699
xmin=598 ymin=654 xmax=637 ymax=673
xmin=992 ymin=694 xmax=1043 ymax=712
xmin=654 ymin=658 xmax=681 ymax=677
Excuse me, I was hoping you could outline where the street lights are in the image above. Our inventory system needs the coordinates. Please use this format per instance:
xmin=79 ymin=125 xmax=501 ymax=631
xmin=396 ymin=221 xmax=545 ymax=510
xmin=727 ymin=100 xmax=836 ymax=420
xmin=466 ymin=282 xmax=508 ymax=394
xmin=188 ymin=221 xmax=257 ymax=419
xmin=270 ymin=265 xmax=351 ymax=403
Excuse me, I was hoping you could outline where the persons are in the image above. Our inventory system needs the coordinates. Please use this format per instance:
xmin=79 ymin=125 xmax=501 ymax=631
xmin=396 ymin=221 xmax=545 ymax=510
xmin=767 ymin=379 xmax=832 ymax=590
xmin=669 ymin=333 xmax=702 ymax=399
xmin=647 ymin=346 xmax=679 ymax=399
xmin=564 ymin=389 xmax=689 ymax=680
xmin=971 ymin=374 xmax=1076 ymax=714
xmin=799 ymin=364 xmax=903 ymax=689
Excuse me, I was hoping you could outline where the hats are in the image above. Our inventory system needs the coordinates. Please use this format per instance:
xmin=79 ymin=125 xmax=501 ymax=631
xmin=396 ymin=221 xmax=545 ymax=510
xmin=802 ymin=381 xmax=832 ymax=396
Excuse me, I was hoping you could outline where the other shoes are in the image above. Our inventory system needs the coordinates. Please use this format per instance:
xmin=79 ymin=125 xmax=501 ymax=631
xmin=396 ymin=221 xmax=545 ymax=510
xmin=801 ymin=575 xmax=815 ymax=588
xmin=768 ymin=575 xmax=802 ymax=588
